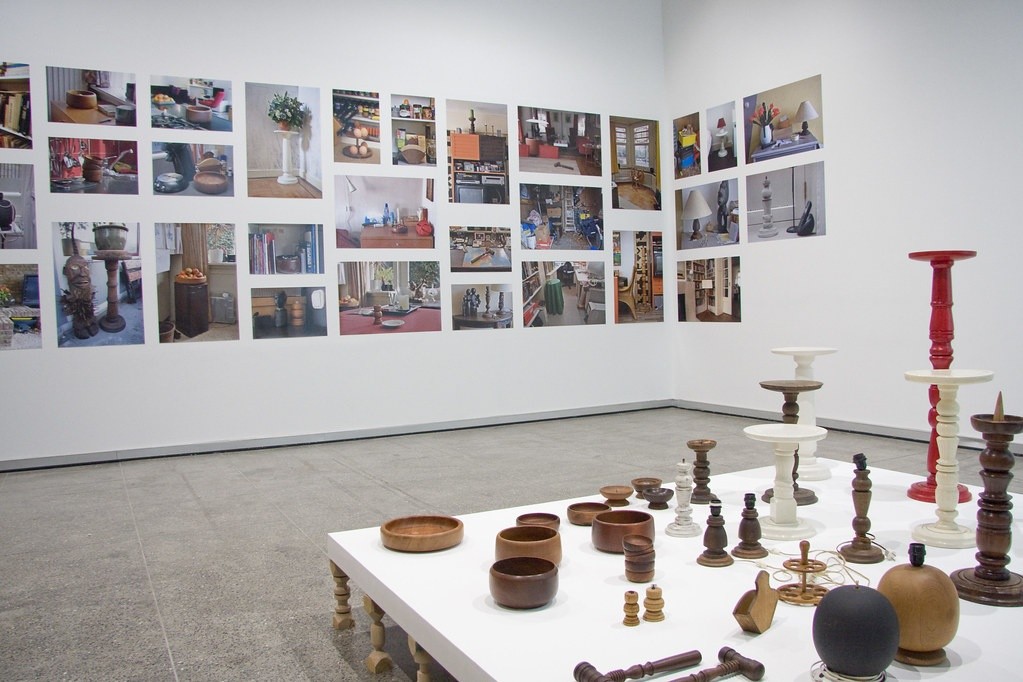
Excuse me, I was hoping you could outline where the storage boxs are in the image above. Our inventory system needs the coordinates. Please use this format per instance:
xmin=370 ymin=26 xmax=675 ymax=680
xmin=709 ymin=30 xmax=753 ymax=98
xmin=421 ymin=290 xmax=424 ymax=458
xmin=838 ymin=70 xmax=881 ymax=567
xmin=677 ymin=132 xmax=697 ymax=169
xmin=547 ymin=207 xmax=561 ymax=217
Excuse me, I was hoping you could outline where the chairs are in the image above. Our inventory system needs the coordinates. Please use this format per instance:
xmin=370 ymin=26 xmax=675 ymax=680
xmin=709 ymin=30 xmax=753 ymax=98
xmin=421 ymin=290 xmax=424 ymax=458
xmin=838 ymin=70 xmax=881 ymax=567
xmin=200 ymin=92 xmax=225 ymax=108
xmin=618 ymin=266 xmax=637 ymax=319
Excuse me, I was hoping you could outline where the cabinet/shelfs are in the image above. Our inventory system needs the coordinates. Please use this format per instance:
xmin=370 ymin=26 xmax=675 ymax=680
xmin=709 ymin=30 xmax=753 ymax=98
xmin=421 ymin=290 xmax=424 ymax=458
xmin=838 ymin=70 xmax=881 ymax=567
xmin=450 ymin=132 xmax=508 ymax=204
xmin=649 ymin=232 xmax=662 ymax=309
xmin=683 ymin=256 xmax=733 ymax=316
xmin=174 ymin=282 xmax=209 ymax=338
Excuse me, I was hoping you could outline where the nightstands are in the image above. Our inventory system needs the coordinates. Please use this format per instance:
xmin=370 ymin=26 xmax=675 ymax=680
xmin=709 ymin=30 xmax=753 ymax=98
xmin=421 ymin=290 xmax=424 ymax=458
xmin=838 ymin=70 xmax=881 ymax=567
xmin=360 ymin=225 xmax=435 ymax=248
xmin=751 ymin=133 xmax=818 ymax=162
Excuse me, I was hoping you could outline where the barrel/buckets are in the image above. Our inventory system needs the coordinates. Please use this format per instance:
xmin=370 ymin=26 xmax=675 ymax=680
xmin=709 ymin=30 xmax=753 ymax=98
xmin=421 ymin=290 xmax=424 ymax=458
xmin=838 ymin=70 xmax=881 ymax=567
xmin=159 ymin=320 xmax=175 ymax=343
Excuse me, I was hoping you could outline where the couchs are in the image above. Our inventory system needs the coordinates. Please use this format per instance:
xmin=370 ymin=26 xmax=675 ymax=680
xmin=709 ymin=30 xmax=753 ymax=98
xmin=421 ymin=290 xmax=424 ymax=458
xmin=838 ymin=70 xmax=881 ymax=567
xmin=526 ymin=138 xmax=544 ymax=157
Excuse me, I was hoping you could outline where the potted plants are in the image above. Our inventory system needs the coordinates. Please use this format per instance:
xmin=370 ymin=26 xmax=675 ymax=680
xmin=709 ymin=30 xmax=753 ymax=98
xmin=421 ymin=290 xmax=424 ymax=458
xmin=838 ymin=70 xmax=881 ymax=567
xmin=376 ymin=267 xmax=394 ymax=292
xmin=93 ymin=222 xmax=129 ymax=249
xmin=207 ymin=224 xmax=224 ymax=262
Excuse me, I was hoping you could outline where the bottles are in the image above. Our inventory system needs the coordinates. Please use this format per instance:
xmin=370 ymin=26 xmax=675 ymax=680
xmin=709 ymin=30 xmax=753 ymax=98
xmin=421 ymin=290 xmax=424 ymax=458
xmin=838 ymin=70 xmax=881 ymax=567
xmin=331 ymin=88 xmax=380 ymax=122
xmin=290 ymin=300 xmax=303 ymax=326
xmin=383 ymin=202 xmax=388 ymax=224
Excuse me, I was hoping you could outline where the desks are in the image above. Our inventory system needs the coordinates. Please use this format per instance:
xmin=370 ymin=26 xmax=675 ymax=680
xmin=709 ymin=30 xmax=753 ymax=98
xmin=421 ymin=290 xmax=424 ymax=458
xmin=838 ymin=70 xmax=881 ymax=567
xmin=716 ymin=131 xmax=728 ymax=158
xmin=340 ymin=307 xmax=441 ymax=334
xmin=91 ymin=256 xmax=133 ymax=332
xmin=452 ymin=311 xmax=512 ymax=330
xmin=51 ymin=99 xmax=117 ymax=125
xmin=274 ymin=130 xmax=300 ymax=185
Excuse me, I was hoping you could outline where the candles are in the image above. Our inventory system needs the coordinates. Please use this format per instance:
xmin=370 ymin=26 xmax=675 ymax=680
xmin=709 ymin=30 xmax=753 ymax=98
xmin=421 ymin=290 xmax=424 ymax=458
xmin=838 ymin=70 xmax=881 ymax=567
xmin=398 ymin=295 xmax=409 ymax=310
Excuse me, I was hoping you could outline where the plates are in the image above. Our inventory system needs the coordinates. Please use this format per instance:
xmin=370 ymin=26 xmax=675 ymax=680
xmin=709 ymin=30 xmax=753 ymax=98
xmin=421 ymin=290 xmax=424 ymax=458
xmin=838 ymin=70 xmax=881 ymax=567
xmin=381 ymin=515 xmax=463 ymax=551
xmin=380 ymin=319 xmax=405 ymax=328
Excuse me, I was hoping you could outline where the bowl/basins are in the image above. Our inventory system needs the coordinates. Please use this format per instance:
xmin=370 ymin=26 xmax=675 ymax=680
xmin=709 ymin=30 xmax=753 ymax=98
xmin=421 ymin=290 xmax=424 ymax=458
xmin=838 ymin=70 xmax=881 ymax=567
xmin=185 ymin=104 xmax=212 ymax=124
xmin=489 ymin=512 xmax=559 ymax=608
xmin=622 ymin=534 xmax=655 ymax=582
xmin=154 ymin=172 xmax=189 ymax=194
xmin=593 ymin=510 xmax=655 ymax=552
xmin=567 ymin=503 xmax=611 ymax=525
xmin=193 ymin=171 xmax=228 ymax=194
xmin=80 ymin=154 xmax=104 ymax=181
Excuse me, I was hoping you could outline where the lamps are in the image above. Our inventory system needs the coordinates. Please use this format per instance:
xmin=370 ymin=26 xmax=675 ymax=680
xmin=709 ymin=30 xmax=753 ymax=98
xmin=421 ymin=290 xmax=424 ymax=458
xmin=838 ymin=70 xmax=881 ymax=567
xmin=681 ymin=190 xmax=712 ymax=241
xmin=717 ymin=118 xmax=726 ymax=133
xmin=491 ymin=284 xmax=512 ymax=315
xmin=795 ymin=101 xmax=818 ymax=136
xmin=344 ymin=175 xmax=357 ymax=226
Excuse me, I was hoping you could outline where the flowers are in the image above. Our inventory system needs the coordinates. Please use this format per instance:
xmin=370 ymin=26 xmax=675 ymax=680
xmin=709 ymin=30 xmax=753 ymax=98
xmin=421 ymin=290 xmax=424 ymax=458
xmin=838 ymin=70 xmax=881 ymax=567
xmin=750 ymin=102 xmax=779 ymax=126
xmin=269 ymin=92 xmax=304 ymax=129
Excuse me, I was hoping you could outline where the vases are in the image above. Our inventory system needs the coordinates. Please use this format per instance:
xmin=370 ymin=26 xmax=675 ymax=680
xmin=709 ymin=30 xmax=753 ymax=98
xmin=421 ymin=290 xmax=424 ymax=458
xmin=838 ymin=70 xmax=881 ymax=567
xmin=279 ymin=120 xmax=290 ymax=131
xmin=761 ymin=125 xmax=772 ymax=144
